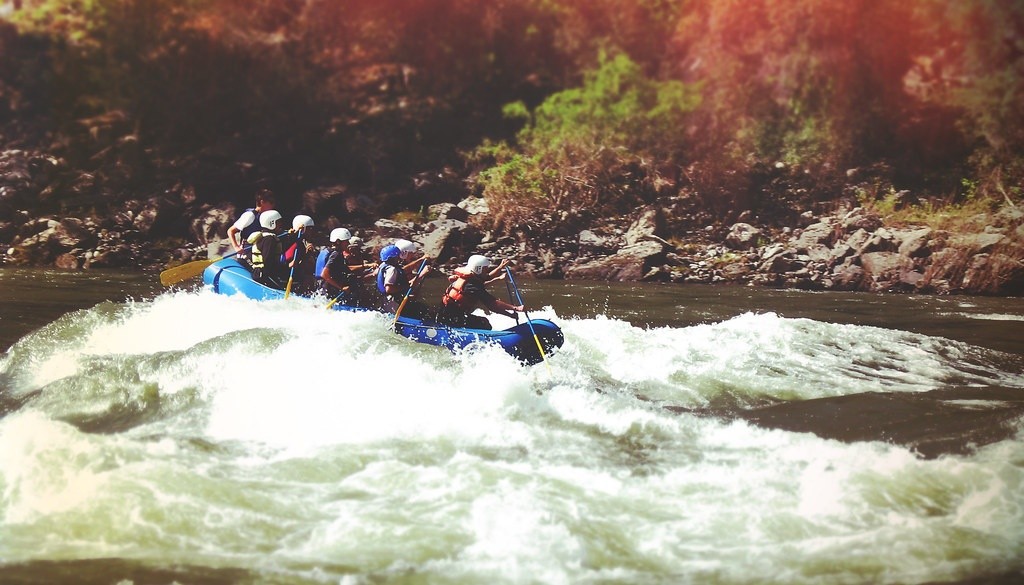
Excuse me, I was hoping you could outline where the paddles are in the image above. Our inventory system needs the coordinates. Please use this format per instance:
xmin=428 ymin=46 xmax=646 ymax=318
xmin=326 ymin=290 xmax=346 ymax=308
xmin=504 ymin=280 xmax=518 ymax=325
xmin=506 ymin=264 xmax=546 ymax=361
xmin=395 ymin=258 xmax=427 ymax=322
xmin=283 ymin=229 xmax=301 ymax=299
xmin=159 ymin=244 xmax=252 ymax=287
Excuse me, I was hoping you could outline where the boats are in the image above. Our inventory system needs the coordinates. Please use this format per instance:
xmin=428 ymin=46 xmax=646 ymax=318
xmin=202 ymin=257 xmax=564 ymax=368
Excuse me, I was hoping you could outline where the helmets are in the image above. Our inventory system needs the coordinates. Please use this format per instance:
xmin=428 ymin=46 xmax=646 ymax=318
xmin=467 ymin=253 xmax=490 ymax=275
xmin=258 ymin=209 xmax=281 ymax=230
xmin=291 ymin=214 xmax=315 ymax=235
xmin=377 ymin=244 xmax=396 ymax=263
xmin=395 ymin=239 xmax=417 ymax=260
xmin=328 ymin=227 xmax=351 ymax=242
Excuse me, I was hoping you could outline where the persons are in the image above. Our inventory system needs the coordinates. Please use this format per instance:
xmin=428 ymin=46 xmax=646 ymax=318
xmin=284 ymin=215 xmax=316 ymax=293
xmin=376 ymin=246 xmax=434 ymax=323
xmin=227 ymin=189 xmax=277 ymax=274
xmin=319 ymin=228 xmax=377 ymax=307
xmin=252 ymin=210 xmax=306 ymax=296
xmin=395 ymin=240 xmax=432 ymax=287
xmin=442 ymin=254 xmax=527 ymax=332
xmin=343 ymin=237 xmax=377 ymax=281
xmin=443 ymin=273 xmax=518 ymax=319
